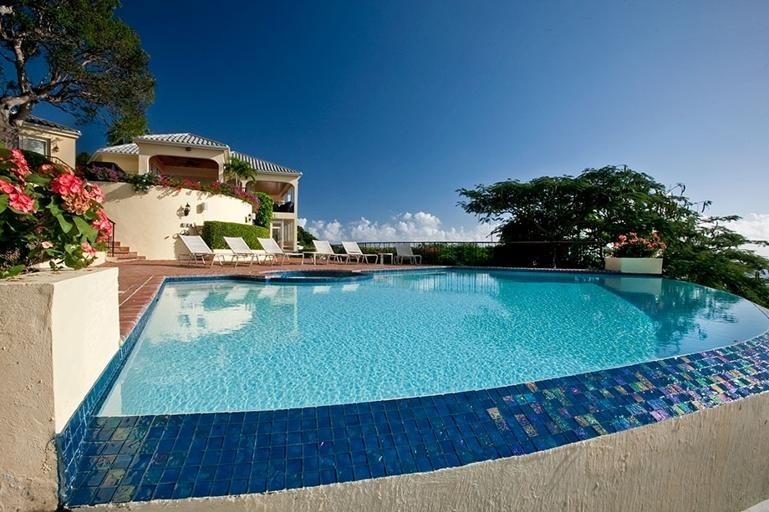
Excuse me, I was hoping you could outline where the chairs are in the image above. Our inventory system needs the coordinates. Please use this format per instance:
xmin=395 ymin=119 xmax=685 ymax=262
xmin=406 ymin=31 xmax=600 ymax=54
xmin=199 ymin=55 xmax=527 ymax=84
xmin=177 ymin=234 xmax=424 ymax=270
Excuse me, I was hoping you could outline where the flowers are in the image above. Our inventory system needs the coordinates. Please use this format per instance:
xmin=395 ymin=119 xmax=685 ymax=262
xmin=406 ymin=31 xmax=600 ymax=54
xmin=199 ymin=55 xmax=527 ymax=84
xmin=85 ymin=159 xmax=260 ymax=214
xmin=604 ymin=227 xmax=666 ymax=258
xmin=0 ymin=143 xmax=115 ymax=280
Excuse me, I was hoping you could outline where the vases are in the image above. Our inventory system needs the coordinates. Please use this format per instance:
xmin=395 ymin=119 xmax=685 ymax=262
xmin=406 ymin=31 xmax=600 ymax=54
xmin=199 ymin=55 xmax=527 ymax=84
xmin=604 ymin=255 xmax=663 ymax=275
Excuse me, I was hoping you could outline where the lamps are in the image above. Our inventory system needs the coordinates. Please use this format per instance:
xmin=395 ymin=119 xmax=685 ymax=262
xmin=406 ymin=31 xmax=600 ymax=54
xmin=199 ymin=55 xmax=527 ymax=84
xmin=184 ymin=201 xmax=191 ymax=216
